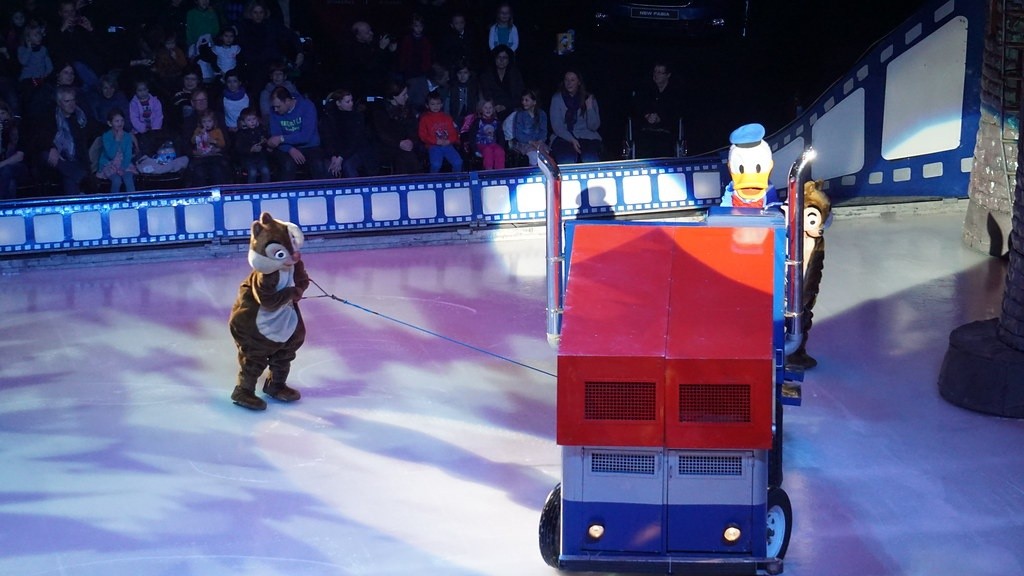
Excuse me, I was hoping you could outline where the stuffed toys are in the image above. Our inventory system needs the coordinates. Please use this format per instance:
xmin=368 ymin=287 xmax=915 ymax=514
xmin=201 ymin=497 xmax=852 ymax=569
xmin=780 ymin=180 xmax=835 ymax=367
xmin=226 ymin=213 xmax=310 ymax=410
xmin=718 ymin=123 xmax=781 ymax=213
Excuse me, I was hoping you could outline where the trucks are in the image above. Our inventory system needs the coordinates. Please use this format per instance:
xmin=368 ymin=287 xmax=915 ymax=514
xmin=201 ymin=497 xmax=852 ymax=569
xmin=530 ymin=141 xmax=819 ymax=576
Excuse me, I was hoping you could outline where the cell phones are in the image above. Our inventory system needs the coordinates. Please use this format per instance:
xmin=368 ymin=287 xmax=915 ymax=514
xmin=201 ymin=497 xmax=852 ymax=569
xmin=67 ymin=16 xmax=83 ymax=27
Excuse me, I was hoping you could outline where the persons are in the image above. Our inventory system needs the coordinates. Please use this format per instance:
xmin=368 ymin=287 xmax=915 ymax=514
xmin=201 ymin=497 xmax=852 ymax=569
xmin=190 ymin=111 xmax=227 ymax=172
xmin=129 ymin=80 xmax=163 ymax=157
xmin=468 ymin=98 xmax=506 ymax=172
xmin=95 ymin=108 xmax=137 ymax=193
xmin=548 ymin=68 xmax=603 ymax=166
xmin=180 ymin=89 xmax=233 ymax=184
xmin=0 ymin=105 xmax=28 ymax=202
xmin=322 ymin=91 xmax=380 ymax=178
xmin=1 ymin=1 xmax=587 ymax=119
xmin=88 ymin=75 xmax=134 ymax=132
xmin=37 ymin=87 xmax=92 ymax=197
xmin=229 ymin=107 xmax=271 ymax=183
xmin=267 ymin=87 xmax=321 ymax=183
xmin=417 ymin=91 xmax=463 ymax=174
xmin=376 ymin=81 xmax=422 ymax=176
xmin=511 ymin=88 xmax=549 ymax=167
xmin=219 ymin=70 xmax=251 ymax=165
xmin=630 ymin=61 xmax=678 ymax=158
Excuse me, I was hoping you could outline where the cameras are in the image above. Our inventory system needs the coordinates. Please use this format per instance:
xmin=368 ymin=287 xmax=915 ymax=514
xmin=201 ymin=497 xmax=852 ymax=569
xmin=0 ymin=116 xmax=21 ymax=131
xmin=379 ymin=32 xmax=400 ymax=45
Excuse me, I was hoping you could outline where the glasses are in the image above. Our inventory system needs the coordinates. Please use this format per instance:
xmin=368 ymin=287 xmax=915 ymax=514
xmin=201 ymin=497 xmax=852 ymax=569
xmin=653 ymin=70 xmax=670 ymax=76
xmin=192 ymin=98 xmax=208 ymax=103
